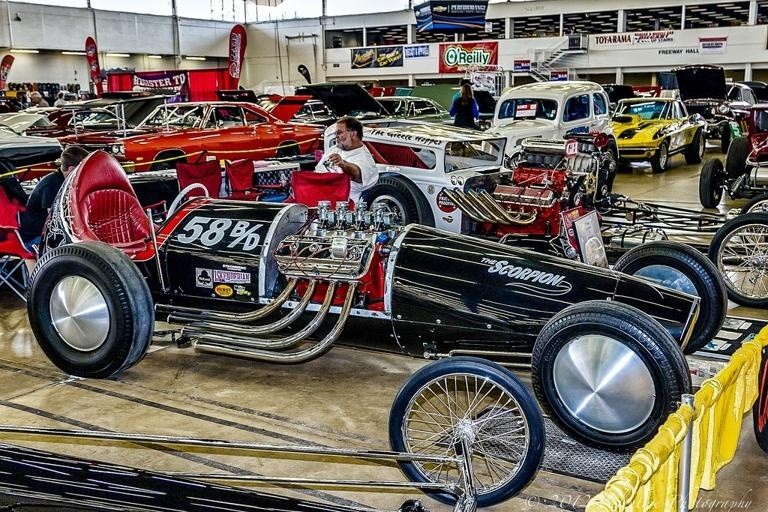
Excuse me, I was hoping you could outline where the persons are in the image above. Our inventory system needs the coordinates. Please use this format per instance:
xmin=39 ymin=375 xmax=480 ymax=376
xmin=19 ymin=147 xmax=89 ymax=252
xmin=31 ymin=91 xmax=87 ymax=107
xmin=282 ymin=117 xmax=378 ymax=211
xmin=449 ymin=84 xmax=479 ymax=128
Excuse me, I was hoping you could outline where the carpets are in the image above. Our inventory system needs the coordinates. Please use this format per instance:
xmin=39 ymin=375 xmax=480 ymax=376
xmin=433 ymin=405 xmax=644 ymax=485
xmin=691 ymin=316 xmax=768 ymax=362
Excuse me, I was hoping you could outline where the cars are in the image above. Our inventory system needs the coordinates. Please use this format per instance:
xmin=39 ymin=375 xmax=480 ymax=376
xmin=0 ymin=64 xmax=766 ymax=451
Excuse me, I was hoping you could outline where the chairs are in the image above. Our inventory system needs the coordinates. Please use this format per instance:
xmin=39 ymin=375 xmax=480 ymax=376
xmin=176 ymin=160 xmax=264 ymax=208
xmin=79 ymin=189 xmax=158 ymax=253
xmin=283 ymin=171 xmax=351 ymax=209
xmin=225 ymin=158 xmax=286 ymax=203
